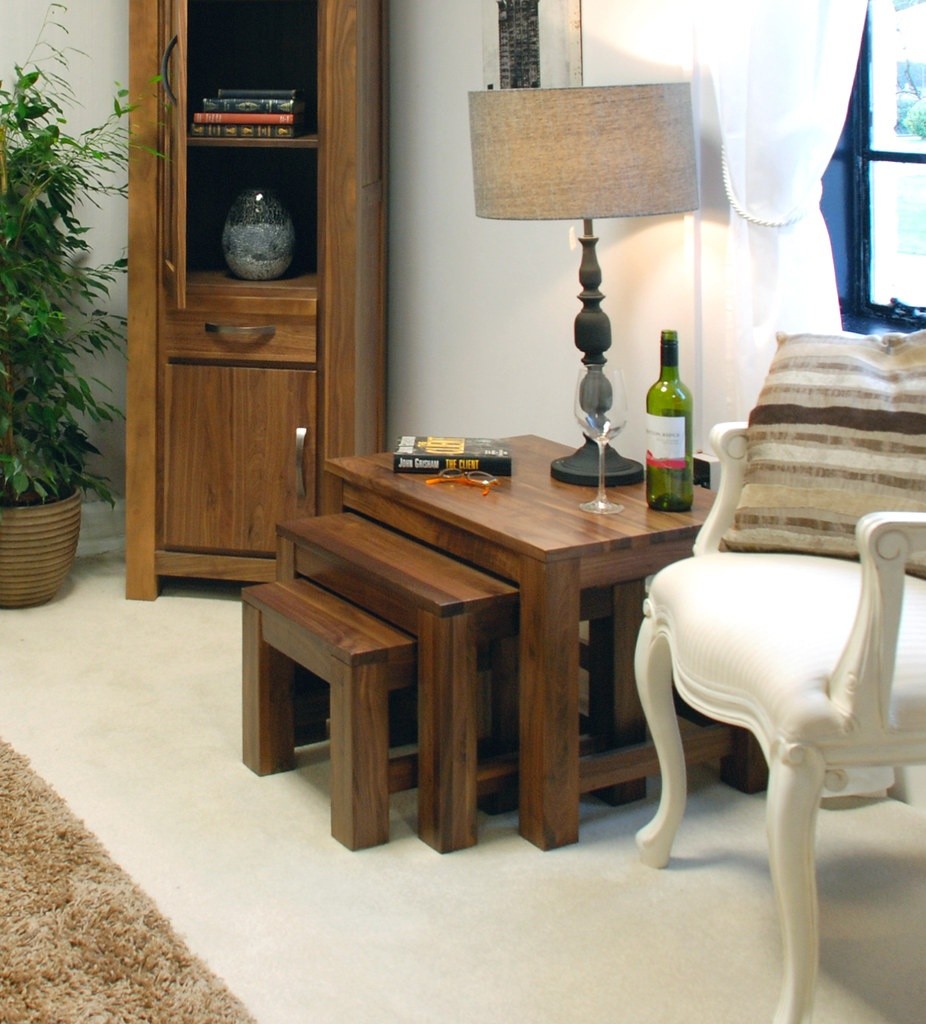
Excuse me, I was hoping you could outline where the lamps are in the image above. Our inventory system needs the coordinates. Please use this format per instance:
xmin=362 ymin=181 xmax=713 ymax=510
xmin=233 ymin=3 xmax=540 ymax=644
xmin=470 ymin=81 xmax=701 ymax=488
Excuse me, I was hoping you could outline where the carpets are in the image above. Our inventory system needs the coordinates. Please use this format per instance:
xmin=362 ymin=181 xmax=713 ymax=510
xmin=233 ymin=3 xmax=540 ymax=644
xmin=1 ymin=737 xmax=264 ymax=1022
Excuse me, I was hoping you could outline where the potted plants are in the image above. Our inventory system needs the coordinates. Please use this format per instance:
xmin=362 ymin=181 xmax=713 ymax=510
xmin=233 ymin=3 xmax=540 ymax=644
xmin=2 ymin=0 xmax=169 ymax=611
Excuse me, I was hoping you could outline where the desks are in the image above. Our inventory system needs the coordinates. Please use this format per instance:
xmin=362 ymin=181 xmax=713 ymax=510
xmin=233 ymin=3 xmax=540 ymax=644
xmin=320 ymin=435 xmax=723 ymax=855
xmin=268 ymin=514 xmax=647 ymax=855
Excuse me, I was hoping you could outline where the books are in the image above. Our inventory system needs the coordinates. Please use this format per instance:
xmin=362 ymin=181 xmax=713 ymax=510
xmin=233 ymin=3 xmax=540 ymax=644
xmin=188 ymin=82 xmax=309 ymax=137
xmin=390 ymin=432 xmax=514 ymax=479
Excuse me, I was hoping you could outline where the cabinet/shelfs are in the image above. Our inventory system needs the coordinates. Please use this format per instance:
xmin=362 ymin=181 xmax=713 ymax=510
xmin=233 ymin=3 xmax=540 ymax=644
xmin=126 ymin=1 xmax=387 ymax=605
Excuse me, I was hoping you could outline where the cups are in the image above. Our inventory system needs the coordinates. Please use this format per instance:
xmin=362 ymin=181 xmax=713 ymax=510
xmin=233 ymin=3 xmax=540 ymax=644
xmin=572 ymin=364 xmax=628 ymax=514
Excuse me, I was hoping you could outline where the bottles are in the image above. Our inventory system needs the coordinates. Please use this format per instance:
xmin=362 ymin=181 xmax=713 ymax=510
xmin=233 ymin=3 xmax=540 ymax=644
xmin=645 ymin=329 xmax=694 ymax=514
xmin=222 ymin=188 xmax=294 ymax=280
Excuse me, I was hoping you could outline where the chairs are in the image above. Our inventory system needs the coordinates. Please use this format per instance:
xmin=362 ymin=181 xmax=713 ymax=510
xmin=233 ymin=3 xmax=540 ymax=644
xmin=632 ymin=419 xmax=925 ymax=1022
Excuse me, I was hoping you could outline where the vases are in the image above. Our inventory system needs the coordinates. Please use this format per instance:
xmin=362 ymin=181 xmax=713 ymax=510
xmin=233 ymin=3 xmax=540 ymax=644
xmin=219 ymin=183 xmax=298 ymax=283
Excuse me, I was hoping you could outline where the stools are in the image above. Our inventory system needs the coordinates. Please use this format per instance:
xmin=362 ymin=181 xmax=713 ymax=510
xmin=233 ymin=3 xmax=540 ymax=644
xmin=240 ymin=576 xmax=512 ymax=851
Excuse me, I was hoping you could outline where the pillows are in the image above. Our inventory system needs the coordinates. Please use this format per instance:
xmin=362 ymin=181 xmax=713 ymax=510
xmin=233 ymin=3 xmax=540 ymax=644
xmin=717 ymin=323 xmax=926 ymax=581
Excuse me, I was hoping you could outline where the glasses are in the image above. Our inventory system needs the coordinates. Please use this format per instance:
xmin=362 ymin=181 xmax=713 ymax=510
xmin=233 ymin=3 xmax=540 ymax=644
xmin=427 ymin=468 xmax=501 ymax=496
